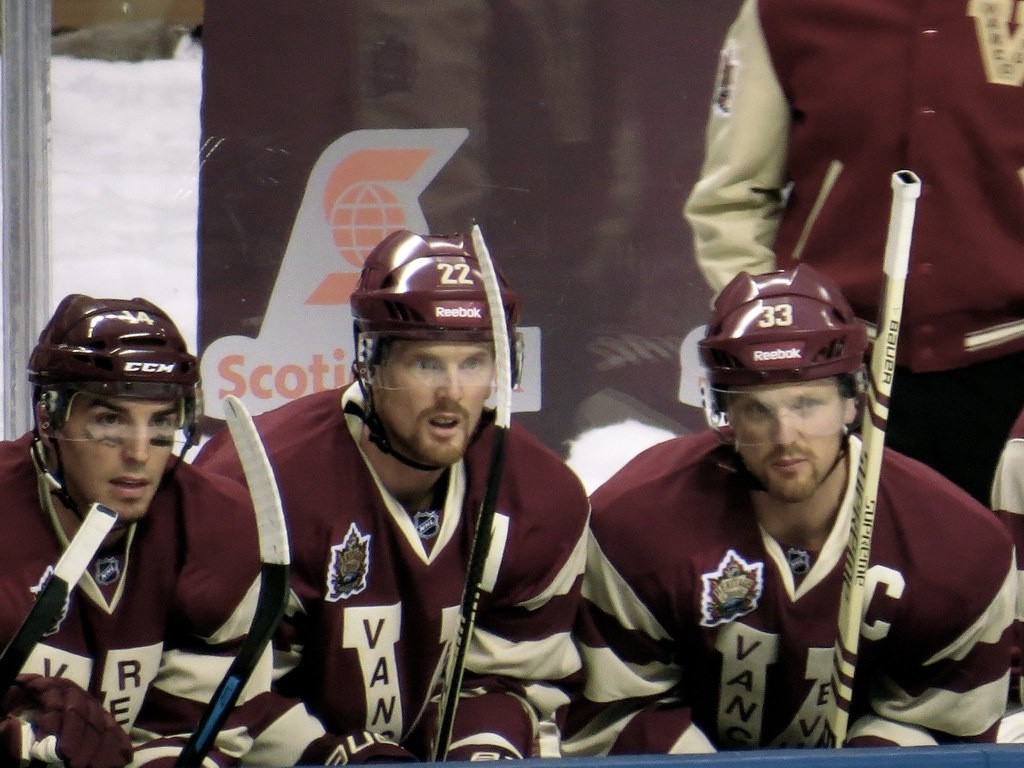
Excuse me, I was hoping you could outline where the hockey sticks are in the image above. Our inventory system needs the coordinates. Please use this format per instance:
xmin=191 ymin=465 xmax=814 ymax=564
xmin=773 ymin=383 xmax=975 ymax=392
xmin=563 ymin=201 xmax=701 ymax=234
xmin=1 ymin=500 xmax=121 ymax=706
xmin=173 ymin=392 xmax=294 ymax=768
xmin=416 ymin=218 xmax=516 ymax=766
xmin=817 ymin=167 xmax=922 ymax=752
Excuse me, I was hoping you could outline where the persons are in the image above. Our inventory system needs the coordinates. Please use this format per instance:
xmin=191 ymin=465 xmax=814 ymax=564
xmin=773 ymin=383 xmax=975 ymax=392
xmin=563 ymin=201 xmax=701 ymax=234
xmin=683 ymin=0 xmax=1024 ymax=510
xmin=551 ymin=263 xmax=1015 ymax=755
xmin=189 ymin=228 xmax=592 ymax=766
xmin=0 ymin=293 xmax=274 ymax=768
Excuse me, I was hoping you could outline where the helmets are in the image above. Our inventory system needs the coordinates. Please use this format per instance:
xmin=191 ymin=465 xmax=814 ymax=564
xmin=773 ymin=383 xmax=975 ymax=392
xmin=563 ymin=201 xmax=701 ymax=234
xmin=349 ymin=228 xmax=525 ymax=344
xmin=27 ymin=294 xmax=205 ymax=391
xmin=695 ymin=261 xmax=870 ymax=387
